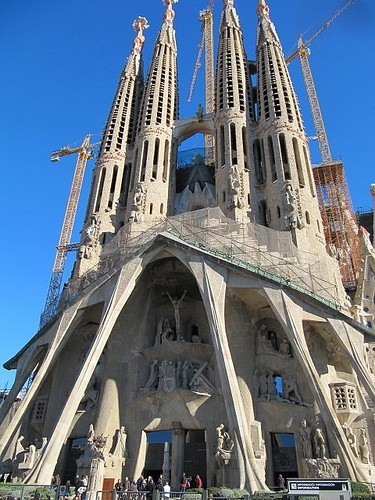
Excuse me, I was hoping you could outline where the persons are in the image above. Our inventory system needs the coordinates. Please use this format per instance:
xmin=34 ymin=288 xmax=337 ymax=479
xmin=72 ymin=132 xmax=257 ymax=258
xmin=23 ymin=442 xmax=36 ymax=464
xmin=215 ymin=423 xmax=225 ymax=457
xmin=52 ymin=474 xmax=88 ymax=500
xmin=130 ymin=475 xmax=164 ymax=500
xmin=87 ymin=423 xmax=95 ymax=444
xmin=277 ymin=474 xmax=285 ymax=488
xmin=114 ymin=425 xmax=129 ymax=458
xmin=166 ymin=290 xmax=187 ymax=341
xmin=115 ymin=479 xmax=123 ymax=491
xmin=347 ymin=427 xmax=357 ymax=458
xmin=163 ymin=481 xmax=171 ymax=500
xmin=299 ymin=416 xmax=330 ymax=461
xmin=179 ymin=472 xmax=187 ymax=496
xmin=252 ymin=326 xmax=313 ymax=408
xmin=143 ymin=316 xmax=213 ymax=394
xmin=196 ymin=474 xmax=202 ymax=488
xmin=201 ymin=473 xmax=207 ymax=489
xmin=186 ymin=473 xmax=196 ymax=490
xmin=123 ymin=476 xmax=130 ymax=495
xmin=359 ymin=429 xmax=370 ymax=464
